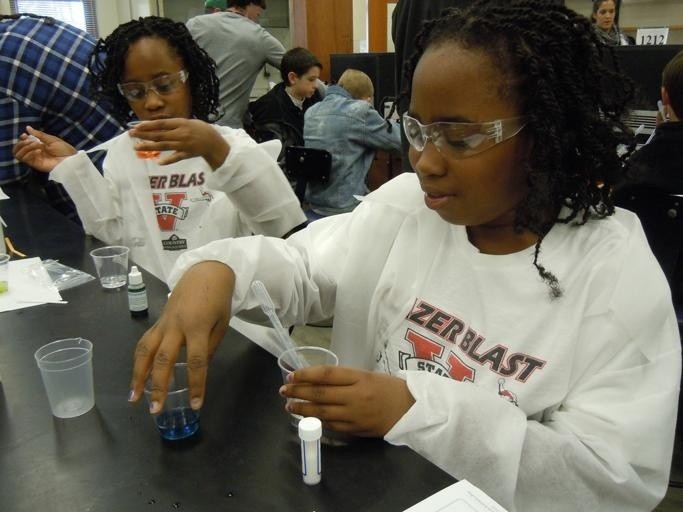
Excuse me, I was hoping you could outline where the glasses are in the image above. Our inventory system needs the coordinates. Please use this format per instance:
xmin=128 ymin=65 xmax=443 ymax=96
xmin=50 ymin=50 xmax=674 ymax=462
xmin=402 ymin=111 xmax=526 ymax=159
xmin=118 ymin=68 xmax=189 ymax=103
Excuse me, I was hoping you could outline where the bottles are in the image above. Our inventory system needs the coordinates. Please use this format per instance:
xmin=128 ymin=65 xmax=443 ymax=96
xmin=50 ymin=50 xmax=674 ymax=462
xmin=125 ymin=264 xmax=150 ymax=321
xmin=298 ymin=417 xmax=325 ymax=486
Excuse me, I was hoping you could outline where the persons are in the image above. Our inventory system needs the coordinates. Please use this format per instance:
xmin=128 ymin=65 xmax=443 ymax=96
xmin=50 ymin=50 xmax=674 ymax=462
xmin=0 ymin=12 xmax=137 ymax=221
xmin=184 ymin=0 xmax=287 ymax=126
xmin=624 ymin=51 xmax=683 ymax=207
xmin=390 ymin=0 xmax=473 ymax=173
xmin=250 ymin=46 xmax=322 ymax=143
xmin=301 ymin=68 xmax=405 ymax=215
xmin=126 ymin=0 xmax=683 ymax=510
xmin=12 ymin=16 xmax=308 ymax=361
xmin=591 ymin=0 xmax=631 ymax=44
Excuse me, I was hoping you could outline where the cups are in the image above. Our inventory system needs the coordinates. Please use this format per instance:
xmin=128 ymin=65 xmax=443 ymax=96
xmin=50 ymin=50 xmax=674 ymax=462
xmin=144 ymin=359 xmax=202 ymax=443
xmin=0 ymin=251 xmax=10 ymax=298
xmin=127 ymin=120 xmax=164 ymax=160
xmin=90 ymin=244 xmax=131 ymax=290
xmin=278 ymin=343 xmax=338 ymax=421
xmin=33 ymin=337 xmax=97 ymax=422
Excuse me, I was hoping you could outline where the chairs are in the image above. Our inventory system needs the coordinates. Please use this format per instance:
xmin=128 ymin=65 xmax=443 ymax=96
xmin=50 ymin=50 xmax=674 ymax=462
xmin=284 ymin=146 xmax=332 ymax=227
